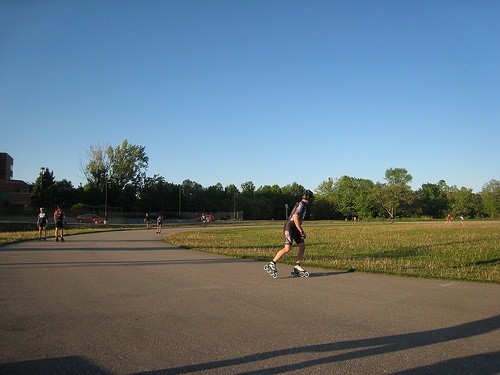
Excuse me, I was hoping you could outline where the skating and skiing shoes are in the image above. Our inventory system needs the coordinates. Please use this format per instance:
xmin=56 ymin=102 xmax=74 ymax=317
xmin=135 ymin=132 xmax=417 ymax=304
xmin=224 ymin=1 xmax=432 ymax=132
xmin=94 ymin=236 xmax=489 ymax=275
xmin=38 ymin=236 xmax=65 ymax=242
xmin=291 ymin=265 xmax=309 ymax=278
xmin=264 ymin=261 xmax=278 ymax=279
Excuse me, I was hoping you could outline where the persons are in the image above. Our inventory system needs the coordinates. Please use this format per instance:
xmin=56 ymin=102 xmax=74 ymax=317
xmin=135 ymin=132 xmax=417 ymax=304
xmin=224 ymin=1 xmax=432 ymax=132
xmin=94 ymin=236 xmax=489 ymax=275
xmin=156 ymin=215 xmax=163 ymax=234
xmin=201 ymin=212 xmax=208 ymax=228
xmin=144 ymin=213 xmax=151 ymax=230
xmin=37 ymin=207 xmax=49 ymax=241
xmin=264 ymin=190 xmax=315 ymax=278
xmin=445 ymin=213 xmax=464 ymax=225
xmin=53 ymin=204 xmax=66 ymax=242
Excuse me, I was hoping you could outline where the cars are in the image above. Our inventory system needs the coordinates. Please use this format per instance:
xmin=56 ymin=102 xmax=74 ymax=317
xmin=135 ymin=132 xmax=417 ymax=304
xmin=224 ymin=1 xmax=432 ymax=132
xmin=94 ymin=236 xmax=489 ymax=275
xmin=194 ymin=215 xmax=215 ymax=223
xmin=76 ymin=213 xmax=104 ymax=225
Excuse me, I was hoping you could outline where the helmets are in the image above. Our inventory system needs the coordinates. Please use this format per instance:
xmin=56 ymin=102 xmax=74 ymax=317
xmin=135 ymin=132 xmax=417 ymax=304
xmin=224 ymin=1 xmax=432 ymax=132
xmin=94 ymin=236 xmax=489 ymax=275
xmin=303 ymin=190 xmax=313 ymax=198
